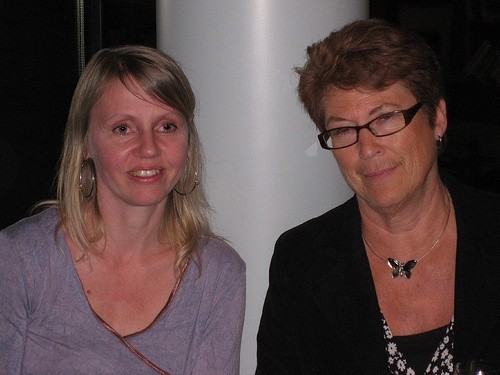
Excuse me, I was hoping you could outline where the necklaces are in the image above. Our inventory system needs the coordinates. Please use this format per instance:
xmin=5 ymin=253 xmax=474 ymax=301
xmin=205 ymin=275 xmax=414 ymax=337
xmin=362 ymin=190 xmax=450 ymax=280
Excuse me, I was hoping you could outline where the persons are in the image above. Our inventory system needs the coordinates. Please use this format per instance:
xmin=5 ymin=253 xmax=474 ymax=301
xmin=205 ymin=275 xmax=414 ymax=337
xmin=254 ymin=18 xmax=500 ymax=375
xmin=0 ymin=46 xmax=246 ymax=375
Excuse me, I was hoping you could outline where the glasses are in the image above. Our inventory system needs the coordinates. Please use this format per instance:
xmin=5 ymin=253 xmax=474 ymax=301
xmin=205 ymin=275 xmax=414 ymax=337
xmin=318 ymin=102 xmax=424 ymax=150
